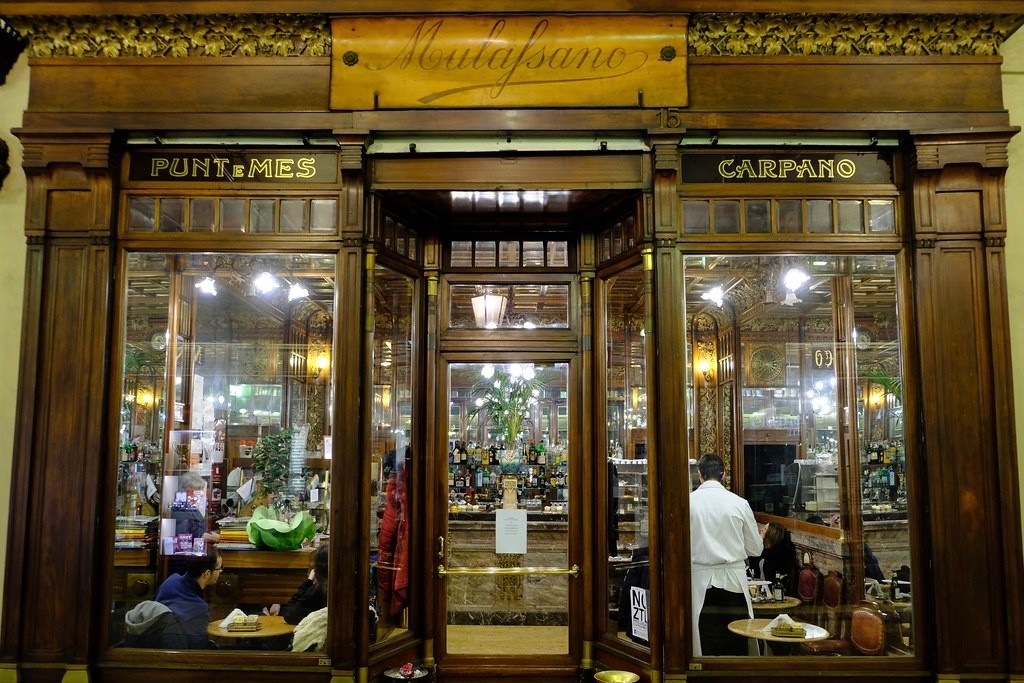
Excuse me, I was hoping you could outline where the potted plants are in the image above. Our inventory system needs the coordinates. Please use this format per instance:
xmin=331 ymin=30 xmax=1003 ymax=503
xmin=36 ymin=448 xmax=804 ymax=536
xmin=463 ymin=366 xmax=559 ymax=475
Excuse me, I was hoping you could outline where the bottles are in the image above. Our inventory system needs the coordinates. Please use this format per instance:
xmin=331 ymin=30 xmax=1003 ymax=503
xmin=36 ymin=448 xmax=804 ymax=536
xmin=493 ymin=498 xmax=501 ymax=510
xmin=517 ymin=466 xmax=568 ymax=488
xmin=863 ymin=465 xmax=906 ymax=502
xmin=774 ymin=573 xmax=785 ymax=602
xmin=863 ymin=438 xmax=905 ymax=465
xmin=890 ymin=572 xmax=900 ymax=602
xmin=448 ymin=465 xmax=502 ymax=487
xmin=450 ymin=439 xmax=506 ymax=465
xmin=520 ymin=498 xmax=567 ymax=514
xmin=519 ymin=438 xmax=546 ymax=465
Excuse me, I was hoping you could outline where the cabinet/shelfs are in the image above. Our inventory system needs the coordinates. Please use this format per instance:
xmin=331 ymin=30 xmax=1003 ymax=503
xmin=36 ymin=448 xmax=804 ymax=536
xmin=448 ymin=463 xmax=568 ymax=502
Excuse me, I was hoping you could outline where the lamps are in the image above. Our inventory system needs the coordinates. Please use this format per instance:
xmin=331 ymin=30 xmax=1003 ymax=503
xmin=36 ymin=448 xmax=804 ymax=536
xmin=471 ymin=288 xmax=508 ymax=329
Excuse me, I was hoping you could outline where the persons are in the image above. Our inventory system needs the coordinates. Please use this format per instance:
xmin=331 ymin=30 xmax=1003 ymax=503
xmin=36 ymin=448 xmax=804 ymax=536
xmin=170 ymin=472 xmax=221 ymax=548
xmin=744 ymin=520 xmax=800 ymax=598
xmin=247 ymin=543 xmax=329 ymax=625
xmin=154 ymin=544 xmax=224 ymax=644
xmin=689 ymin=452 xmax=764 ymax=658
xmin=373 ymin=452 xmax=409 ymax=627
xmin=806 ymin=514 xmax=888 ymax=593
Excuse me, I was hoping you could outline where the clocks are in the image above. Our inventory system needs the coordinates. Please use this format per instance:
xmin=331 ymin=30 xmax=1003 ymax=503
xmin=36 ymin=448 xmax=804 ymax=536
xmin=856 ymin=328 xmax=875 ymax=352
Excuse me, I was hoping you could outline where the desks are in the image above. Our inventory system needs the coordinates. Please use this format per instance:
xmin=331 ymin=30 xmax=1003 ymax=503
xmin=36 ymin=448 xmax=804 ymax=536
xmin=208 ymin=615 xmax=297 ymax=649
xmin=729 ymin=617 xmax=830 ymax=655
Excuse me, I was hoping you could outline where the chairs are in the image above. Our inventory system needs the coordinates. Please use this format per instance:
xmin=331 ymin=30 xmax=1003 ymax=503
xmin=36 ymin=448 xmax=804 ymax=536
xmin=799 ymin=600 xmax=879 ymax=655
xmin=799 ymin=564 xmax=843 ymax=639
xmin=126 ymin=601 xmax=187 ymax=650
xmin=838 ymin=606 xmax=888 ymax=657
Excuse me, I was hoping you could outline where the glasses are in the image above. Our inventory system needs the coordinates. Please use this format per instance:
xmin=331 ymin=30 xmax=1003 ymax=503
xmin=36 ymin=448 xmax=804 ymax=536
xmin=203 ymin=565 xmax=224 ymax=574
